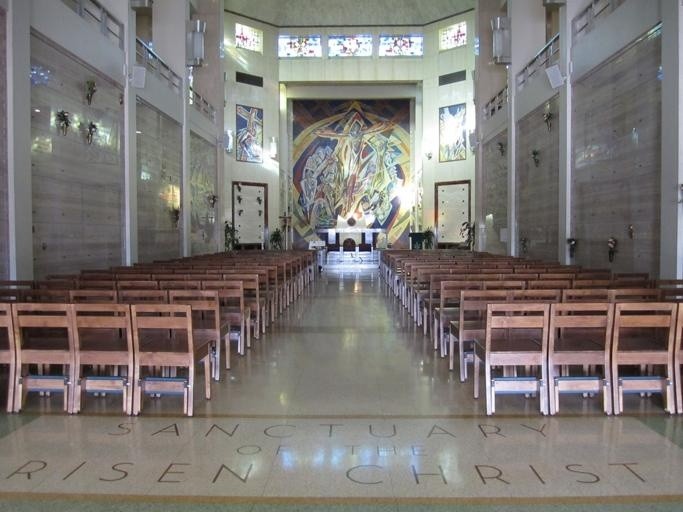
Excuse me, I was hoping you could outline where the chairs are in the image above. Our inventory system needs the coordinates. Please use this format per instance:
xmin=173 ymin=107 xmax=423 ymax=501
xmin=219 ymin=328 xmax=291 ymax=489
xmin=378 ymin=249 xmax=683 ymax=418
xmin=1 ymin=250 xmax=319 ymax=415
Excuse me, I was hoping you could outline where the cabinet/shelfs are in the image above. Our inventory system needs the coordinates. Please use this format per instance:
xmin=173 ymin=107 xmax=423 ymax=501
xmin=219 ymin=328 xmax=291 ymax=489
xmin=315 ymin=226 xmax=385 ymax=251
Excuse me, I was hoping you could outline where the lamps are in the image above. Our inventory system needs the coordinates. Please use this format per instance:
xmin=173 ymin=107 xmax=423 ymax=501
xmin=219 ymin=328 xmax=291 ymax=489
xmin=185 ymin=20 xmax=207 ymax=67
xmin=490 ymin=16 xmax=512 ymax=65
